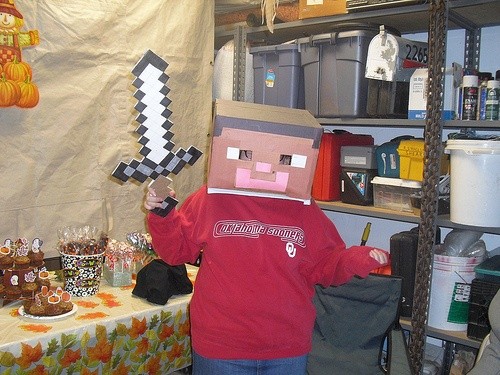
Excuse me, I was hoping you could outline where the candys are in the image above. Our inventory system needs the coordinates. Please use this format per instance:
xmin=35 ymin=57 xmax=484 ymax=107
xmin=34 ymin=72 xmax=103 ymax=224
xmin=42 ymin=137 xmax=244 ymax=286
xmin=59 ymin=231 xmax=157 ymax=273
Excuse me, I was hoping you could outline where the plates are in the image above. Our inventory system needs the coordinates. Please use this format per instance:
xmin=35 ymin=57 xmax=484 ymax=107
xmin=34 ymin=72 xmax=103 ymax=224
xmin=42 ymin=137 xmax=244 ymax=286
xmin=18 ymin=304 xmax=78 ymax=320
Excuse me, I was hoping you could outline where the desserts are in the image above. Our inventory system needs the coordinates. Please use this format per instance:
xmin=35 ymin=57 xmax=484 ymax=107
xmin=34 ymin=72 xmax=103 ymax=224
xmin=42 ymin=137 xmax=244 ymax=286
xmin=23 ymin=291 xmax=73 ymax=315
xmin=0 ymin=249 xmax=51 ymax=299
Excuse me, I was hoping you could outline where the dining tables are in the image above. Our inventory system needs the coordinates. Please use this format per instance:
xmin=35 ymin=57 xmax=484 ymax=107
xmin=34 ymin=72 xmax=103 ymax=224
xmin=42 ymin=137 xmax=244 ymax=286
xmin=0 ymin=262 xmax=200 ymax=375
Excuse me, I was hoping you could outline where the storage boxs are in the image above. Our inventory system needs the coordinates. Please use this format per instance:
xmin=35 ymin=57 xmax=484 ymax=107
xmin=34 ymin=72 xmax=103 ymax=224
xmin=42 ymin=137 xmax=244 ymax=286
xmin=311 ymin=130 xmax=451 ymax=215
xmin=248 ymin=44 xmax=305 ymax=111
xmin=297 ymin=22 xmax=402 ymax=118
xmin=408 ymin=62 xmax=462 ymax=120
xmin=207 ymin=96 xmax=324 ymax=201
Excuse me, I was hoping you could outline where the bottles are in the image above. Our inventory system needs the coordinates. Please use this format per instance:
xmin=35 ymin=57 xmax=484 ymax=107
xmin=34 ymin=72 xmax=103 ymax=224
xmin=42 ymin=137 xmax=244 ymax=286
xmin=460 ymin=71 xmax=500 ymax=121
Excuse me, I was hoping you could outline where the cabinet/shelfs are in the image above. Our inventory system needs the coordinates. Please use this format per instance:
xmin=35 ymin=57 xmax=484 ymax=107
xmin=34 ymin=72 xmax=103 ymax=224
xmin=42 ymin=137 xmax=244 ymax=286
xmin=214 ymin=0 xmax=500 ymax=375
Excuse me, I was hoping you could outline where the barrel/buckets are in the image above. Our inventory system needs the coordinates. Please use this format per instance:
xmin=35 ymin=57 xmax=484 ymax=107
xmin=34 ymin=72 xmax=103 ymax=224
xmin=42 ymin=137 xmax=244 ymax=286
xmin=427 ymin=244 xmax=484 ymax=330
xmin=58 ymin=250 xmax=106 ymax=298
xmin=445 ymin=139 xmax=499 ymax=228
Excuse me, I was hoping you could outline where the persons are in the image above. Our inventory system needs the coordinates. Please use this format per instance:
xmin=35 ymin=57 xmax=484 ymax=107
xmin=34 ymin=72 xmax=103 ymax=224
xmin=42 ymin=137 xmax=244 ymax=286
xmin=111 ymin=50 xmax=390 ymax=375
